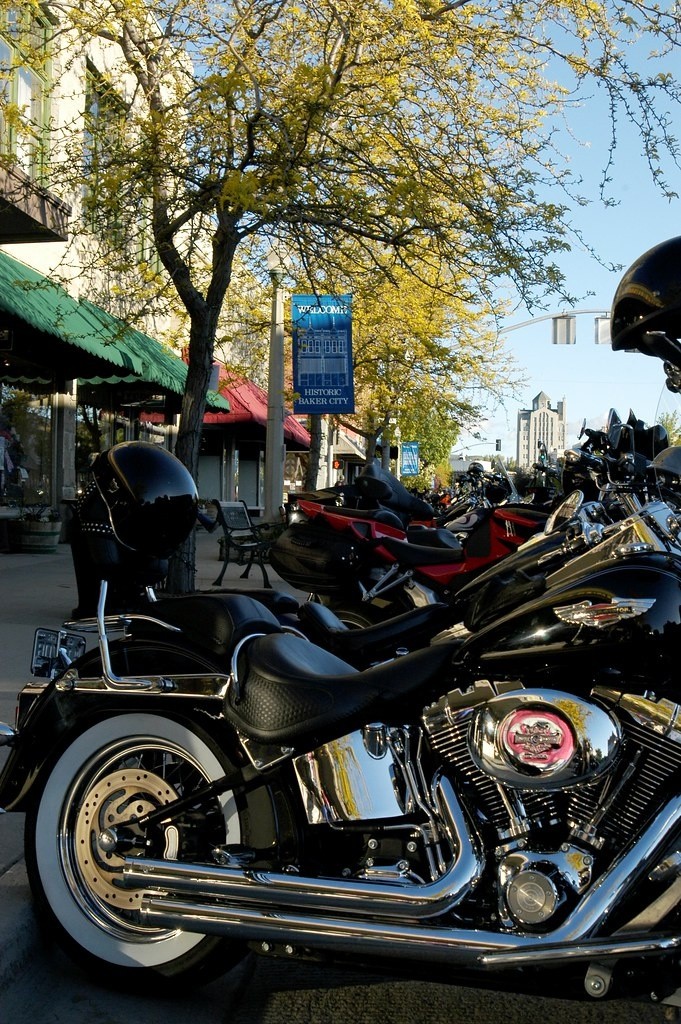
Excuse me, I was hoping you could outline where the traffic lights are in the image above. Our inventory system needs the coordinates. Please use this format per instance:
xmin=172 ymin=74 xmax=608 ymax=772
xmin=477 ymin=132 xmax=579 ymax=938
xmin=540 ymin=450 xmax=545 ymax=459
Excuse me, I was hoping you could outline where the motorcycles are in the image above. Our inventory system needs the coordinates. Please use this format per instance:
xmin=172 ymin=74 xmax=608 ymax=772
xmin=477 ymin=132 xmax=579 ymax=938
xmin=0 ymin=328 xmax=681 ymax=1001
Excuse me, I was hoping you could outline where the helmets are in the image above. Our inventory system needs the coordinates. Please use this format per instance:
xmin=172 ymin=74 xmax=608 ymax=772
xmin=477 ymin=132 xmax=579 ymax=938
xmin=647 ymin=444 xmax=681 ymax=479
xmin=607 ymin=236 xmax=681 ymax=349
xmin=466 ymin=461 xmax=485 ymax=474
xmin=93 ymin=437 xmax=198 ymax=559
